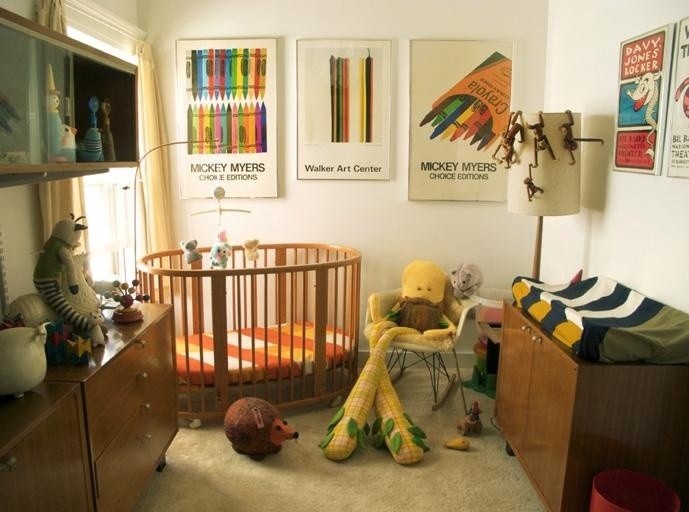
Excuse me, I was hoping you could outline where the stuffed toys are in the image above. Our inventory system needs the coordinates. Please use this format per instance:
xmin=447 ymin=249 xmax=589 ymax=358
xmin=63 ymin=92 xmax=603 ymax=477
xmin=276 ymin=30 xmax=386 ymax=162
xmin=492 ymin=109 xmax=604 ymax=203
xmin=242 ymin=239 xmax=261 ymax=260
xmin=181 ymin=238 xmax=200 ymax=264
xmin=225 ymin=397 xmax=300 ymax=462
xmin=208 ymin=240 xmax=231 ymax=270
xmin=217 ymin=229 xmax=226 ymax=240
xmin=459 ymin=400 xmax=483 ymax=435
xmin=443 ymin=436 xmax=470 ymax=450
xmin=317 ymin=259 xmax=463 ymax=463
xmin=33 ymin=213 xmax=105 ymax=345
xmin=448 ymin=262 xmax=485 ymax=298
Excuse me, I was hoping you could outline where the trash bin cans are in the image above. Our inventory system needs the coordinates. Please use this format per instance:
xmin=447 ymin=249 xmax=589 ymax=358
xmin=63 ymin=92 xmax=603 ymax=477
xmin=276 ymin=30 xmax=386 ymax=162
xmin=589 ymin=468 xmax=681 ymax=512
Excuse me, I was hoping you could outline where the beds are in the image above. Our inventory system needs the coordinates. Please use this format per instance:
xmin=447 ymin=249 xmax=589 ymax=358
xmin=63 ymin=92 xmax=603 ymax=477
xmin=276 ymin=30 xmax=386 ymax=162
xmin=138 ymin=245 xmax=361 ymax=421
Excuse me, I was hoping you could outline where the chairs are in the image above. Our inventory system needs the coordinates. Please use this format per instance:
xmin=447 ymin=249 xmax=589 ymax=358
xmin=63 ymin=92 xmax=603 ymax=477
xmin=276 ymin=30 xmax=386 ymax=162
xmin=364 ymin=289 xmax=479 ymax=410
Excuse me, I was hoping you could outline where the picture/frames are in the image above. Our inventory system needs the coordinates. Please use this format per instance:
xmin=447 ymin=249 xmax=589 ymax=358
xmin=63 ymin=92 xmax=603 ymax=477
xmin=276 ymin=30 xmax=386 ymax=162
xmin=612 ymin=16 xmax=689 ymax=179
xmin=407 ymin=38 xmax=514 ymax=202
xmin=295 ymin=38 xmax=392 ymax=182
xmin=174 ymin=38 xmax=278 ymax=204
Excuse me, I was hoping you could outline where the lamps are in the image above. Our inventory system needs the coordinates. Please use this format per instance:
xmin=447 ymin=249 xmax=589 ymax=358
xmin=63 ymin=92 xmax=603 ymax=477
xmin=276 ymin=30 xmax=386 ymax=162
xmin=509 ymin=113 xmax=582 ymax=282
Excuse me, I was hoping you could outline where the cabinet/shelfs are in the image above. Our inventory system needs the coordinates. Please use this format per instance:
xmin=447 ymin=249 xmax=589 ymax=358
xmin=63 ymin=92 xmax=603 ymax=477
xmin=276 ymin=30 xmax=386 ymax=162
xmin=0 ymin=9 xmax=141 ymax=180
xmin=0 ymin=382 xmax=94 ymax=512
xmin=493 ymin=303 xmax=689 ymax=512
xmin=45 ymin=302 xmax=180 ymax=512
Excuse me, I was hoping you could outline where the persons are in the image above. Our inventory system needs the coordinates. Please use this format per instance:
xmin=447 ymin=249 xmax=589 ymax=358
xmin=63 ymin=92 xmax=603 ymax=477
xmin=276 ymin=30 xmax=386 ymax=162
xmin=33 ymin=62 xmax=118 ymax=163
